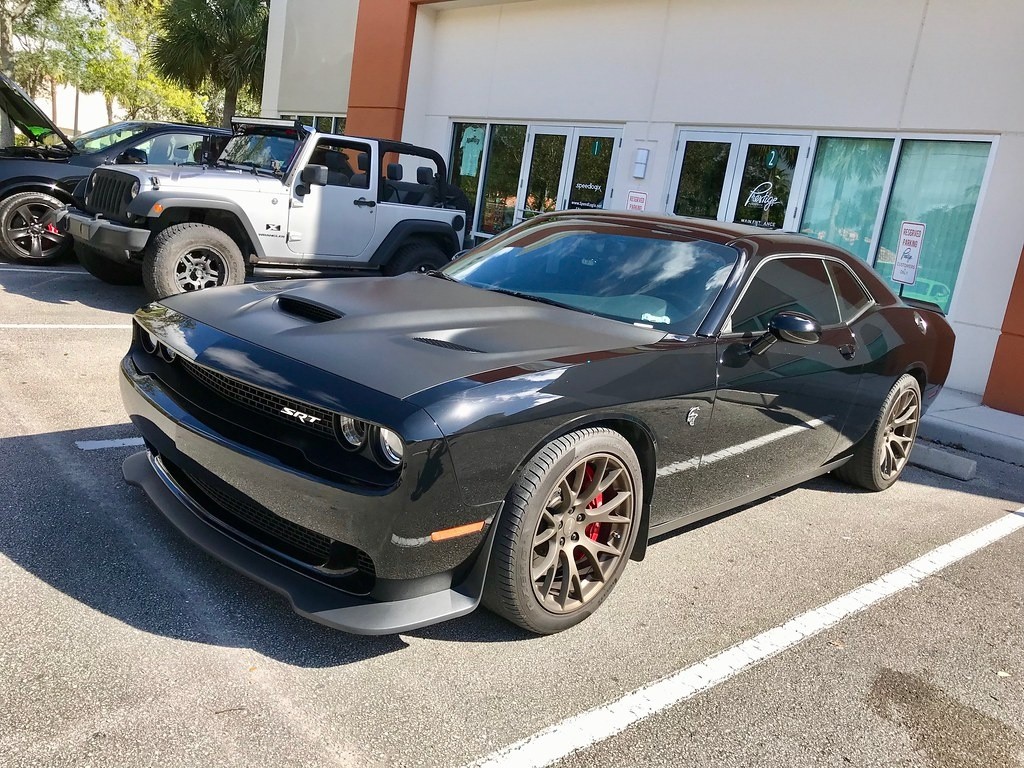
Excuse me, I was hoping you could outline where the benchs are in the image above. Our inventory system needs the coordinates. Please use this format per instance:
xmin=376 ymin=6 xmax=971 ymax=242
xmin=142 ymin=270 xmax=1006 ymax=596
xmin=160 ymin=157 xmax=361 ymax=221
xmin=381 ymin=163 xmax=440 ymax=207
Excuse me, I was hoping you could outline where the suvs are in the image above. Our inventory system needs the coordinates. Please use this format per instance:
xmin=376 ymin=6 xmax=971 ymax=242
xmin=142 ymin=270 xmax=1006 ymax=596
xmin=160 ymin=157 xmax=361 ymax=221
xmin=48 ymin=115 xmax=469 ymax=303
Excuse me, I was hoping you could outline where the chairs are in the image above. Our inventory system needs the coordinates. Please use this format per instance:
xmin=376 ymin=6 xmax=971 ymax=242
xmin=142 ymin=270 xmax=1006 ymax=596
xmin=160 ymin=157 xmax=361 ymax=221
xmin=320 ymin=150 xmax=349 ymax=185
xmin=346 ymin=153 xmax=369 ymax=188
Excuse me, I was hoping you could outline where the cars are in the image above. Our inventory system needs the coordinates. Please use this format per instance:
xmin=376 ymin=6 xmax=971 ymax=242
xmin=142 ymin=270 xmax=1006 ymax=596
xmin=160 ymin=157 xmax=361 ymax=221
xmin=1 ymin=70 xmax=236 ymax=266
xmin=111 ymin=207 xmax=960 ymax=635
